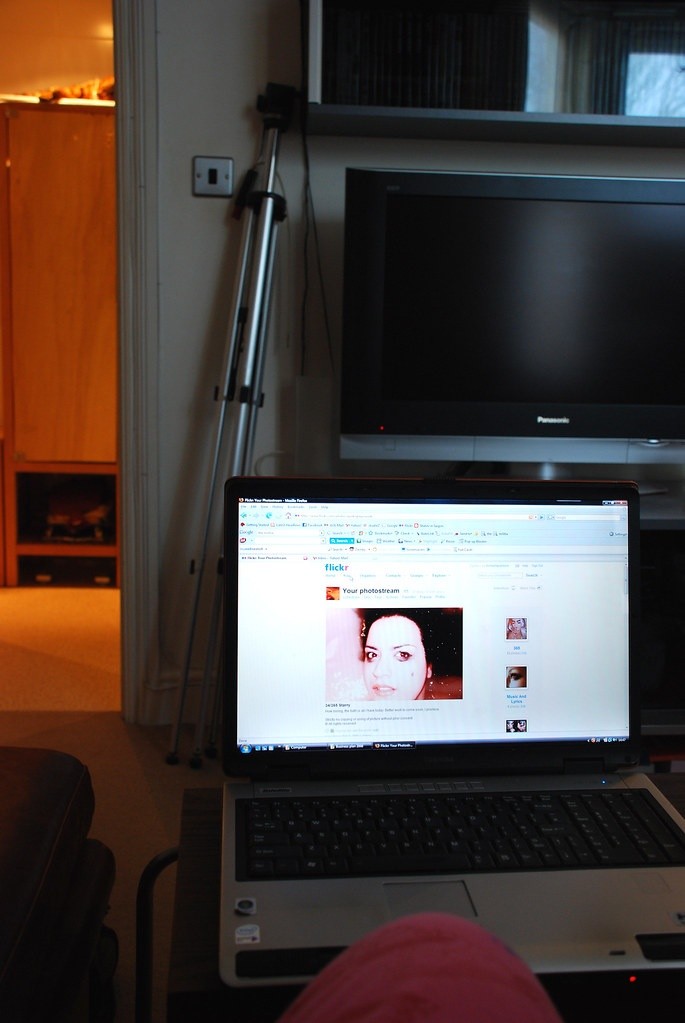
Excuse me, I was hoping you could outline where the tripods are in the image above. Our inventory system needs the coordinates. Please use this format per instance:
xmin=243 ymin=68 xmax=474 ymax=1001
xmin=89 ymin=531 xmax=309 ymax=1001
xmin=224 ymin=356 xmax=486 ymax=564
xmin=164 ymin=83 xmax=298 ymax=772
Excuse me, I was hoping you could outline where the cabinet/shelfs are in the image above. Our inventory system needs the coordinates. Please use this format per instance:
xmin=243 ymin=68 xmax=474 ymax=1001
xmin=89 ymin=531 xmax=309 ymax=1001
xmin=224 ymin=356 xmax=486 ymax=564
xmin=0 ymin=102 xmax=121 ymax=587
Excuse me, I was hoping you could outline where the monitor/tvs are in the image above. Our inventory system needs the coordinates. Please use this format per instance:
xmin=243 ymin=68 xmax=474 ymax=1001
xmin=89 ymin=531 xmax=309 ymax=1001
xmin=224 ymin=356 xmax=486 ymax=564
xmin=337 ymin=166 xmax=685 ymax=495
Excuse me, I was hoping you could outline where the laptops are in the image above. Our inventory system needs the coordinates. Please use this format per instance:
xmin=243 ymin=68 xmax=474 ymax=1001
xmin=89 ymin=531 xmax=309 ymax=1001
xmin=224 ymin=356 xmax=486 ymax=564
xmin=221 ymin=476 xmax=685 ymax=1005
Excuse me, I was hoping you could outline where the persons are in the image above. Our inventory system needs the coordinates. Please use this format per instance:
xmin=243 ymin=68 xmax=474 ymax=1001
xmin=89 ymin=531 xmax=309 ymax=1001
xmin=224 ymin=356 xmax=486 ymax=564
xmin=361 ymin=608 xmax=463 ymax=700
xmin=506 ymin=618 xmax=527 ymax=639
xmin=506 ymin=667 xmax=526 ymax=688
xmin=276 ymin=911 xmax=563 ymax=1023
